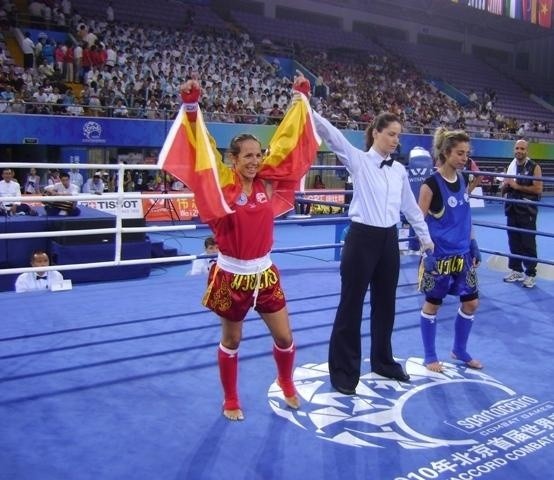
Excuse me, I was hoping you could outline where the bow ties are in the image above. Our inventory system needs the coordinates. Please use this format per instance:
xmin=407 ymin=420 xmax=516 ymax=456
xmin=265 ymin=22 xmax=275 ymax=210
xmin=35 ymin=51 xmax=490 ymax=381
xmin=381 ymin=158 xmax=395 ymax=168
xmin=35 ymin=276 xmax=47 ymax=280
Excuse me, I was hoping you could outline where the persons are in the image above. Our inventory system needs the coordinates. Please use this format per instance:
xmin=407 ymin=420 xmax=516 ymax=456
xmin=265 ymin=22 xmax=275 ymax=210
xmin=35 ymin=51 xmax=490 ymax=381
xmin=0 ymin=0 xmax=554 ymax=220
xmin=12 ymin=247 xmax=64 ymax=291
xmin=407 ymin=124 xmax=489 ymax=375
xmin=289 ymin=86 xmax=437 ymax=398
xmin=188 ymin=237 xmax=221 ymax=274
xmin=493 ymin=137 xmax=545 ymax=287
xmin=413 ymin=148 xmax=481 ymax=296
xmin=174 ymin=74 xmax=328 ymax=425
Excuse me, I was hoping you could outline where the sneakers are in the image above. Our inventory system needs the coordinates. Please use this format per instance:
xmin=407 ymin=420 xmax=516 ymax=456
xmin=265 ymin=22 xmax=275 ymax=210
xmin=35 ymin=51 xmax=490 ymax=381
xmin=522 ymin=276 xmax=537 ymax=288
xmin=504 ymin=271 xmax=524 ymax=282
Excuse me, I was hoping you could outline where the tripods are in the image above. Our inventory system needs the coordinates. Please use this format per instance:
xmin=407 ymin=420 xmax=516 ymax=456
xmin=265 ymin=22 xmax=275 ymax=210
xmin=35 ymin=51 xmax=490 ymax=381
xmin=144 ymin=115 xmax=182 ymax=226
